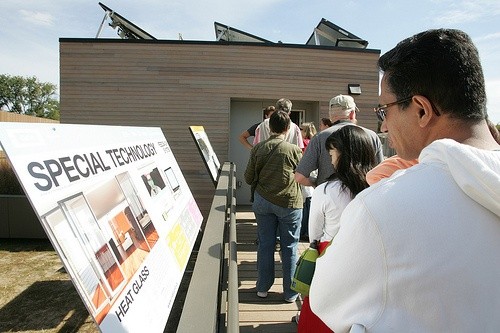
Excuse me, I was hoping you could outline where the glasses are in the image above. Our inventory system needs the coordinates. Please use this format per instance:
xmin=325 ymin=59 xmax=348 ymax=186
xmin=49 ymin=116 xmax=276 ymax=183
xmin=374 ymin=94 xmax=441 ymax=122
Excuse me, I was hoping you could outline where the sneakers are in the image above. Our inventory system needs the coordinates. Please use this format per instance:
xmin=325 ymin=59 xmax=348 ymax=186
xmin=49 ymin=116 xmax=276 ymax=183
xmin=257 ymin=291 xmax=267 ymax=297
xmin=285 ymin=293 xmax=300 ymax=303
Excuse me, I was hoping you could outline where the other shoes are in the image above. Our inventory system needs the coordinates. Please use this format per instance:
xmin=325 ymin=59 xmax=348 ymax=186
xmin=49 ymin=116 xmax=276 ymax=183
xmin=295 ymin=311 xmax=301 ymax=323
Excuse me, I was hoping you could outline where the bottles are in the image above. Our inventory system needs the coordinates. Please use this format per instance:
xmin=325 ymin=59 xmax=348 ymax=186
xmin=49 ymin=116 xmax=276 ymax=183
xmin=290 ymin=239 xmax=319 ymax=296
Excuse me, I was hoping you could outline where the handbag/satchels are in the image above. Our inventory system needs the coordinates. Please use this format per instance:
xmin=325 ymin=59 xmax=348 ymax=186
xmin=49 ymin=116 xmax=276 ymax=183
xmin=290 ymin=239 xmax=319 ymax=296
xmin=249 ymin=182 xmax=257 ymax=203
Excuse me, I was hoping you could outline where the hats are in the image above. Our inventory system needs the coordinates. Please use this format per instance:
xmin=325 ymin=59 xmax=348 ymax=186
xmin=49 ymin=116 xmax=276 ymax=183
xmin=329 ymin=94 xmax=360 ymax=113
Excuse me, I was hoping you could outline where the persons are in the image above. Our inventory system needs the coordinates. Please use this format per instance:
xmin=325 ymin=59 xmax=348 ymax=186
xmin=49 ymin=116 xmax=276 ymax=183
xmin=242 ymin=94 xmax=422 ymax=305
xmin=296 ymin=27 xmax=500 ymax=332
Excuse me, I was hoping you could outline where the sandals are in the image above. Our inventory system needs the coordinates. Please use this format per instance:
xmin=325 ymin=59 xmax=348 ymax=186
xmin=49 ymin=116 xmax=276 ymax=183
xmin=276 ymin=242 xmax=281 ymax=252
